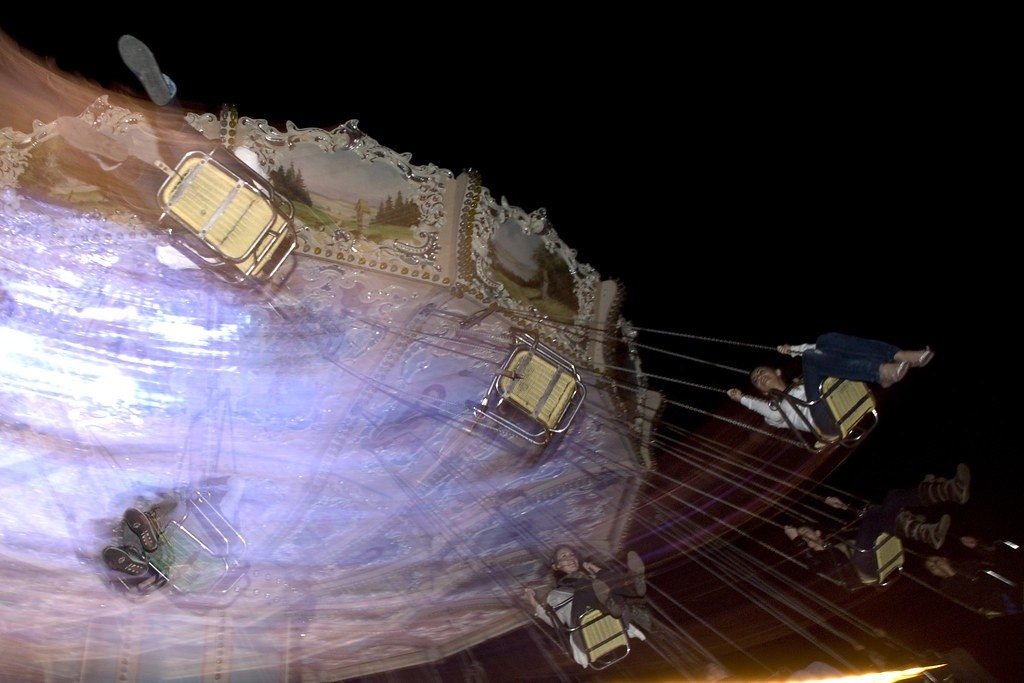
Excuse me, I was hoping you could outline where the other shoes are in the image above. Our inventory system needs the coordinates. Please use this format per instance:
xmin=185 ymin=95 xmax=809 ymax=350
xmin=119 ymin=34 xmax=172 ymax=107
xmin=103 ymin=544 xmax=148 ymax=576
xmin=878 ymin=362 xmax=909 ymax=388
xmin=125 ymin=507 xmax=164 ymax=550
xmin=627 ymin=552 xmax=647 ymax=597
xmin=591 ymin=579 xmax=623 ymax=618
xmin=892 ymin=346 xmax=935 ymax=367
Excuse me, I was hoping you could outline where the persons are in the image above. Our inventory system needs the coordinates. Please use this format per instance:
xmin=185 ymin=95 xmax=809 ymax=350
xmin=517 ymin=543 xmax=647 ymax=631
xmin=57 ymin=35 xmax=297 ymax=291
xmin=725 ymin=333 xmax=935 ymax=444
xmin=93 ymin=491 xmax=178 ymax=594
xmin=782 ymin=462 xmax=971 ymax=593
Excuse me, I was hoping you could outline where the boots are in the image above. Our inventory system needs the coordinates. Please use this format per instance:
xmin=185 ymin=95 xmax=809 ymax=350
xmin=918 ymin=464 xmax=971 ymax=504
xmin=894 ymin=510 xmax=950 ymax=550
